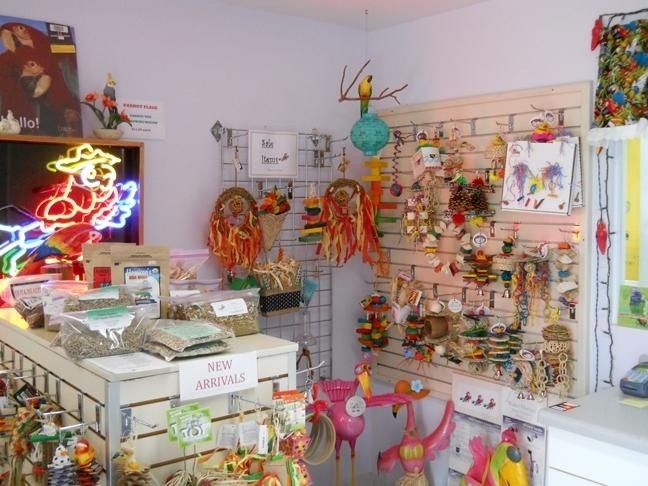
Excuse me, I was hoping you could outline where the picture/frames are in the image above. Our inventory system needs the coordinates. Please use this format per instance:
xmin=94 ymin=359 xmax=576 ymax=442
xmin=247 ymin=131 xmax=298 ymax=179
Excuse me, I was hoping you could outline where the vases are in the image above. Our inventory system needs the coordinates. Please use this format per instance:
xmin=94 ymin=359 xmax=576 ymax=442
xmin=93 ymin=129 xmax=124 ymax=139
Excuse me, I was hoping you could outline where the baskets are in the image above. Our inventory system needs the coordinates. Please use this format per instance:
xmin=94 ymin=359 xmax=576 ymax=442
xmin=259 ymin=270 xmax=300 ymax=317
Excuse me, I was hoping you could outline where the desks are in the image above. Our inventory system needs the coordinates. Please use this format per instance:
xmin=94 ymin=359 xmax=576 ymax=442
xmin=537 ymin=385 xmax=648 ymax=486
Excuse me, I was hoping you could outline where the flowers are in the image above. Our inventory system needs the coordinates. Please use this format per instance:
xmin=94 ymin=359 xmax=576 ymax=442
xmin=79 ymin=72 xmax=134 ymax=128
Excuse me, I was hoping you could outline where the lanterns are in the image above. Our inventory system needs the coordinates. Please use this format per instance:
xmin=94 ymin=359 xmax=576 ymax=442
xmin=350 ymin=112 xmax=390 ymax=157
